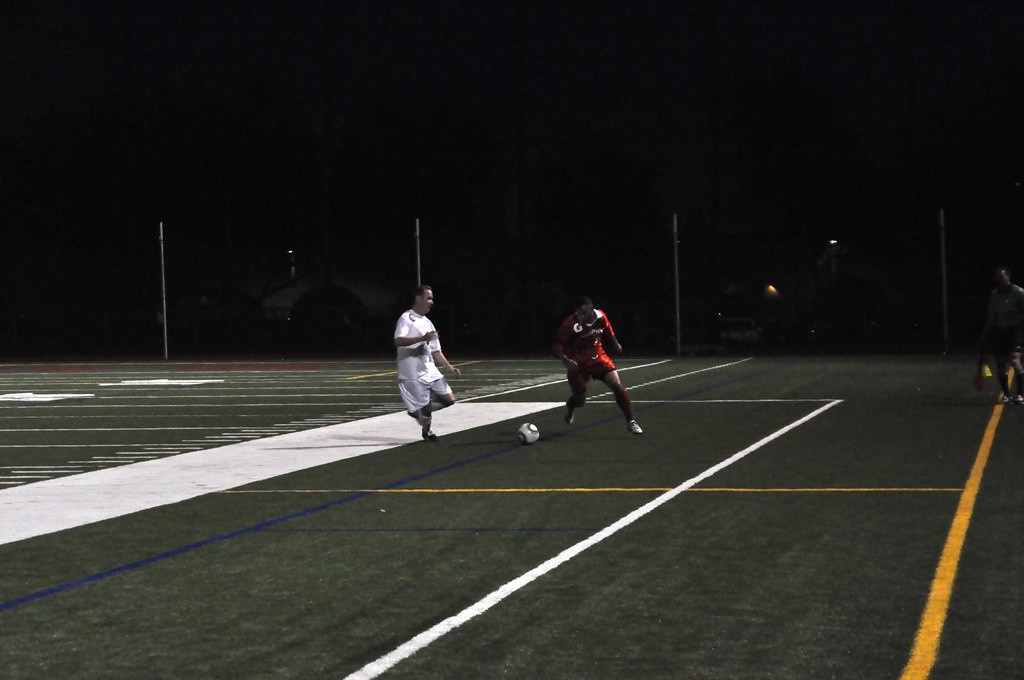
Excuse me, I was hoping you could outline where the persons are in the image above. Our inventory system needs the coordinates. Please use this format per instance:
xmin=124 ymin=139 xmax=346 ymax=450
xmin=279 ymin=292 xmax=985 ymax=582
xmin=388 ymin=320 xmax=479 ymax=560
xmin=977 ymin=264 xmax=1024 ymax=404
xmin=554 ymin=296 xmax=642 ymax=435
xmin=394 ymin=285 xmax=462 ymax=443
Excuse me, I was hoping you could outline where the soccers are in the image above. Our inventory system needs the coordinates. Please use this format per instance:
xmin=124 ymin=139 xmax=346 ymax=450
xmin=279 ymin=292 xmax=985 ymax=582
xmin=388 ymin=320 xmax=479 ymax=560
xmin=517 ymin=422 xmax=540 ymax=445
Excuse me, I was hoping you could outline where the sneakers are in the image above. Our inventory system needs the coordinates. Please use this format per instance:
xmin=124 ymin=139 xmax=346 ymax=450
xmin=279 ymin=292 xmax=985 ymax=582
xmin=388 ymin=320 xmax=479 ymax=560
xmin=421 ymin=428 xmax=439 ymax=442
xmin=1014 ymin=395 xmax=1023 ymax=402
xmin=564 ymin=399 xmax=575 ymax=425
xmin=627 ymin=420 xmax=642 ymax=434
xmin=1002 ymin=395 xmax=1012 ymax=402
